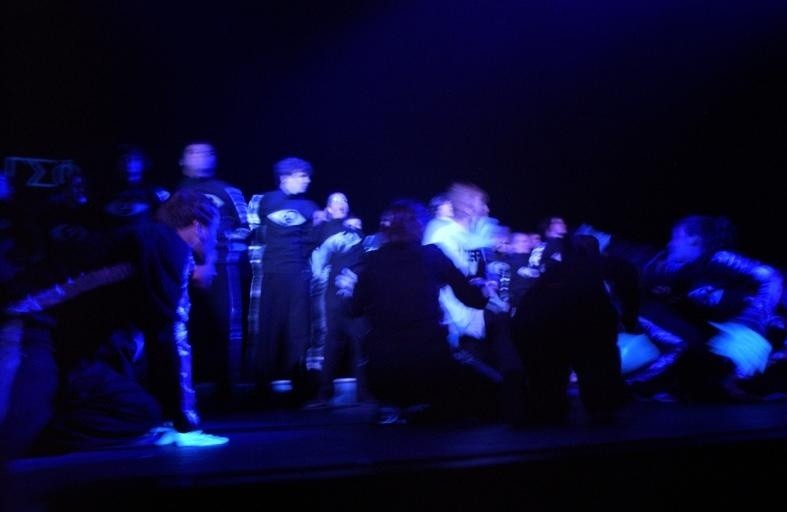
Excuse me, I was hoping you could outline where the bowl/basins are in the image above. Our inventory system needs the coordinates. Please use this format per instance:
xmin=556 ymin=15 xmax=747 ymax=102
xmin=302 ymin=400 xmax=382 ymax=446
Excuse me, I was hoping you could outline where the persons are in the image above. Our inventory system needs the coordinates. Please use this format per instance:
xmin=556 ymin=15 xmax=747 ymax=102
xmin=42 ymin=140 xmax=787 ymax=449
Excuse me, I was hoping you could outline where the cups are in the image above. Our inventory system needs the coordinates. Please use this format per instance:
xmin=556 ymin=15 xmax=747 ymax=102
xmin=332 ymin=377 xmax=357 ymax=404
xmin=271 ymin=380 xmax=292 ymax=391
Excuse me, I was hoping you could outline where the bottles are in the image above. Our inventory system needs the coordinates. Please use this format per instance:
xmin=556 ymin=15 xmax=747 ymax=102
xmin=565 ymin=370 xmax=582 ymax=399
